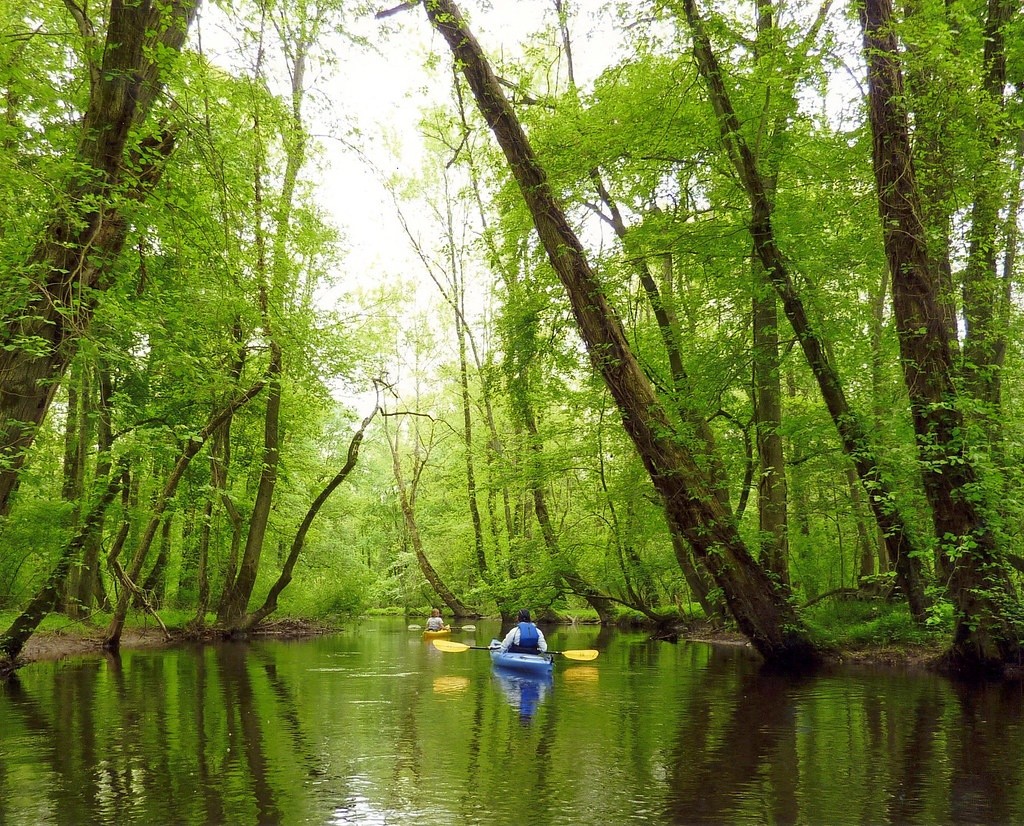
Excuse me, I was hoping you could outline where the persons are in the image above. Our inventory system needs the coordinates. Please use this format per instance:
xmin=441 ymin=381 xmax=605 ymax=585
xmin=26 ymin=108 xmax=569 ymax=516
xmin=426 ymin=609 xmax=449 ymax=632
xmin=501 ymin=610 xmax=548 ymax=655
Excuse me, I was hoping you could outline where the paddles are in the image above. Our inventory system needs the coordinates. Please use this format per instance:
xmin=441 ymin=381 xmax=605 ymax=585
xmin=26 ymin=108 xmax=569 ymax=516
xmin=407 ymin=624 xmax=477 ymax=632
xmin=432 ymin=640 xmax=600 ymax=660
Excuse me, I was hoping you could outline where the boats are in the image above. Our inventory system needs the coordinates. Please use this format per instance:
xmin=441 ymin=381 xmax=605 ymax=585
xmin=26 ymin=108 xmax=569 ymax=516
xmin=422 ymin=624 xmax=451 ymax=641
xmin=489 ymin=639 xmax=556 ymax=677
